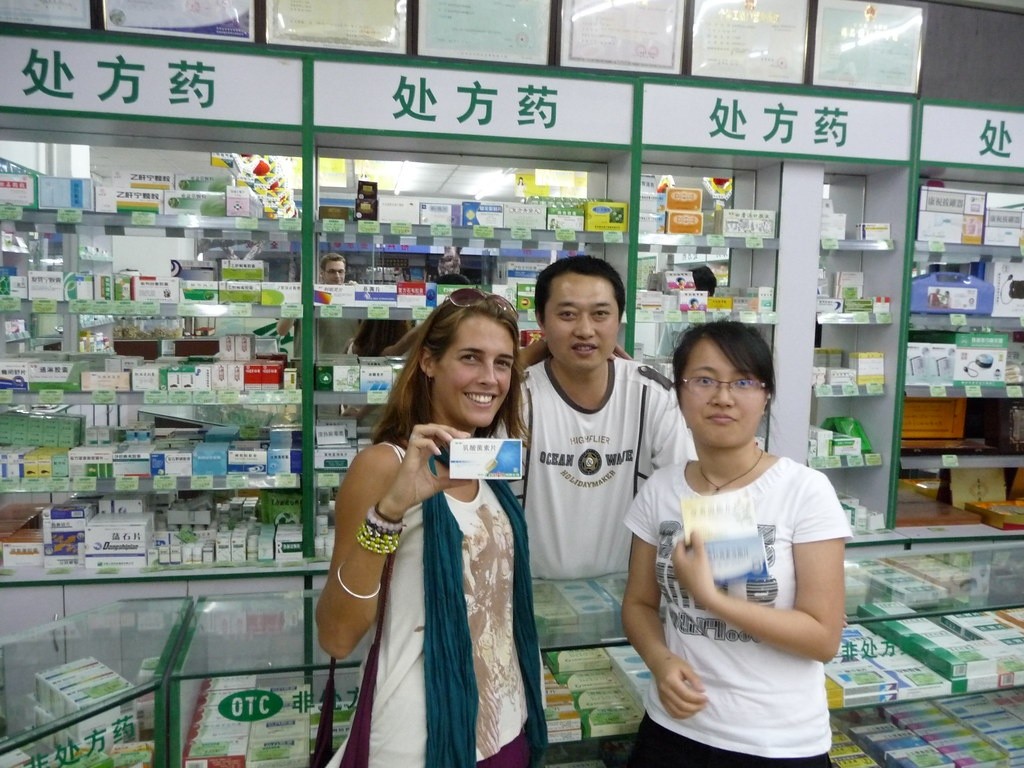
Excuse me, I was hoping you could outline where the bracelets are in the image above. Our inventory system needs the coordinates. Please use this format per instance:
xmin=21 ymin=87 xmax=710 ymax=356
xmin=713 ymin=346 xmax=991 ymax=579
xmin=354 ymin=501 xmax=402 ymax=555
xmin=336 ymin=561 xmax=381 ymax=599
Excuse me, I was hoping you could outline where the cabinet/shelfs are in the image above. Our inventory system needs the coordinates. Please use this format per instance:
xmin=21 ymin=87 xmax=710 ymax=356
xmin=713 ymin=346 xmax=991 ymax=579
xmin=1 ymin=545 xmax=1024 ymax=768
xmin=0 ymin=22 xmax=1024 ymax=734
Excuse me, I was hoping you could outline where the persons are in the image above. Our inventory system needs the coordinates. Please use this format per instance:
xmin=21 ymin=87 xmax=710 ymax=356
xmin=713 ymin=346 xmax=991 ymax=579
xmin=488 ymin=256 xmax=699 ymax=582
xmin=315 ymin=288 xmax=549 ymax=768
xmin=276 ymin=253 xmax=418 ymax=358
xmin=623 ymin=320 xmax=853 ymax=768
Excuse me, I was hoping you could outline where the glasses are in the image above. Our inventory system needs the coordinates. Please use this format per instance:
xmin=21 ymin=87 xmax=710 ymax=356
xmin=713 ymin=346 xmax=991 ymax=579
xmin=443 ymin=288 xmax=520 ymax=319
xmin=680 ymin=376 xmax=766 ymax=395
xmin=328 ymin=268 xmax=345 ymax=274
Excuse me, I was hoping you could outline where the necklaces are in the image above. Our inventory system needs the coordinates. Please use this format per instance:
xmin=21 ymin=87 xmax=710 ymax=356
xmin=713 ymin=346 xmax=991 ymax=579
xmin=700 ymin=447 xmax=764 ymax=493
